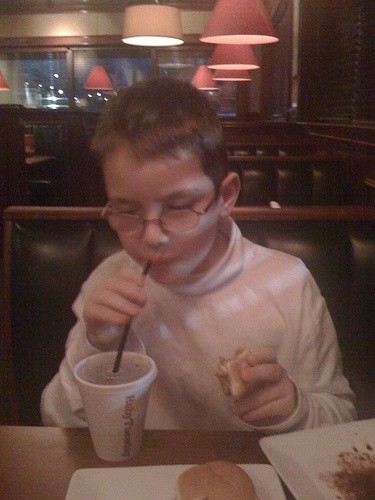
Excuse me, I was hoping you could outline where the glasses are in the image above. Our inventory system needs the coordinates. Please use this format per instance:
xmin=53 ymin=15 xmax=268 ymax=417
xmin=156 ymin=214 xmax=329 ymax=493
xmin=102 ymin=183 xmax=221 ymax=237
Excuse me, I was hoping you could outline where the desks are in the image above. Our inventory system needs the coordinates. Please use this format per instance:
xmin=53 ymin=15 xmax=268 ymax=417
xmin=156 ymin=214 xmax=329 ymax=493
xmin=0 ymin=426 xmax=375 ymax=500
xmin=24 ymin=151 xmax=58 ymax=165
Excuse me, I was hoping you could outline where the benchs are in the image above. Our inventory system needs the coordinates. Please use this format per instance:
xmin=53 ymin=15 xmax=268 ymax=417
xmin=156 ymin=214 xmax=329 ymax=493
xmin=0 ymin=104 xmax=375 ymax=427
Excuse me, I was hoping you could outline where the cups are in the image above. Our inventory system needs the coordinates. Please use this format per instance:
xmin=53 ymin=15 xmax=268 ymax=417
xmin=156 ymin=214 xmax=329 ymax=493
xmin=73 ymin=351 xmax=156 ymax=462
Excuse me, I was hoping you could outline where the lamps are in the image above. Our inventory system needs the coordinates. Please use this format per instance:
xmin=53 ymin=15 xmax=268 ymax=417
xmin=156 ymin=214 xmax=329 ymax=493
xmin=82 ymin=0 xmax=114 ymax=91
xmin=189 ymin=62 xmax=220 ymax=91
xmin=199 ymin=0 xmax=281 ymax=45
xmin=206 ymin=44 xmax=260 ymax=70
xmin=121 ymin=0 xmax=185 ymax=47
xmin=211 ymin=68 xmax=253 ymax=82
xmin=0 ymin=69 xmax=11 ymax=92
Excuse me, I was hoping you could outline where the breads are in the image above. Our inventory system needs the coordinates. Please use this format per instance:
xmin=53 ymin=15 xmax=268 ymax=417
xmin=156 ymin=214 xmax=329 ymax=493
xmin=176 ymin=460 xmax=262 ymax=500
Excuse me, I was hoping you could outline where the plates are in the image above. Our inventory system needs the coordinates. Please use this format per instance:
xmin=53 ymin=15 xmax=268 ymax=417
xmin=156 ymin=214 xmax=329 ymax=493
xmin=259 ymin=418 xmax=375 ymax=500
xmin=65 ymin=464 xmax=287 ymax=500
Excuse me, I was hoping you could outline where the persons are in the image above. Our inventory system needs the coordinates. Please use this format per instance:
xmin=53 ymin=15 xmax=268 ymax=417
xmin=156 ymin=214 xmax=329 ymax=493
xmin=39 ymin=75 xmax=357 ymax=432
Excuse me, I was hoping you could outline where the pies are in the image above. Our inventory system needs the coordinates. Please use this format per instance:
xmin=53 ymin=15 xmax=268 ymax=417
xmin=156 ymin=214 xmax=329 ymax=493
xmin=215 ymin=346 xmax=252 ymax=398
